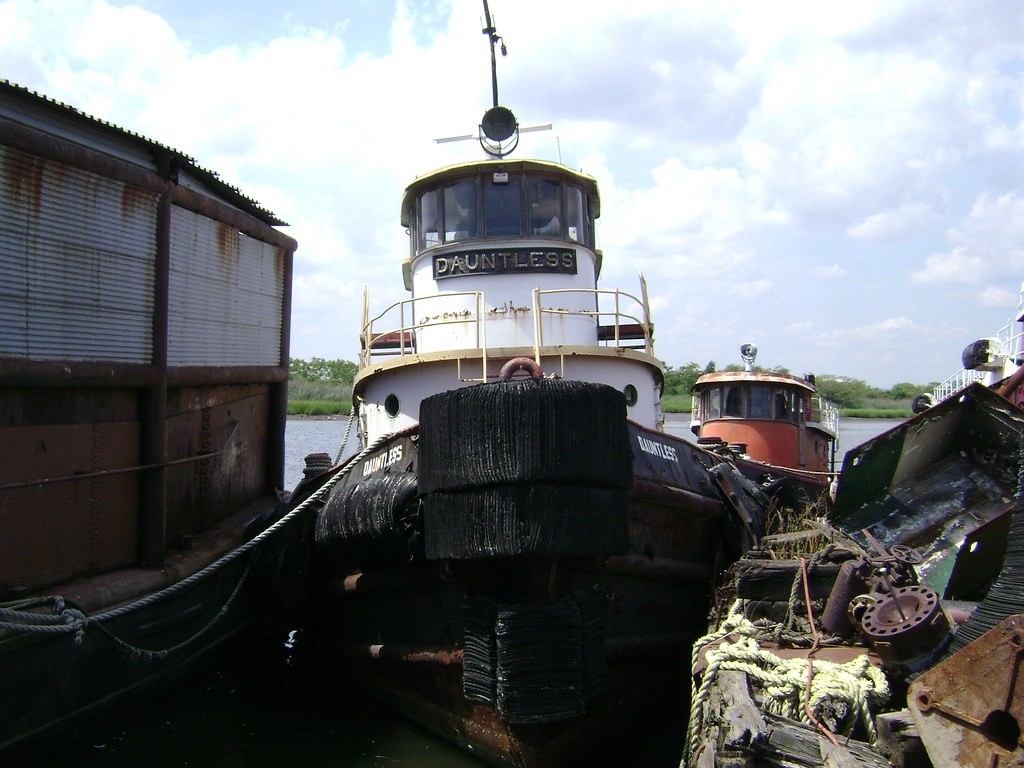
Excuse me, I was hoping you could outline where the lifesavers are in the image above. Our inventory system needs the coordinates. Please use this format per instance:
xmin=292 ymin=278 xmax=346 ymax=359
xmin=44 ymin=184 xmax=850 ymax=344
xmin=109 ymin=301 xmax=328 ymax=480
xmin=498 ymin=357 xmax=544 ymax=381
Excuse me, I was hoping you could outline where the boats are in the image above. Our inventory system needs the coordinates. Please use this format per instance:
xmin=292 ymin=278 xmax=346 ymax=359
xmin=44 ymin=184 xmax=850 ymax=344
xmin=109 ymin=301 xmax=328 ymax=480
xmin=690 ymin=343 xmax=839 ymax=483
xmin=0 ymin=0 xmax=1024 ymax=768
